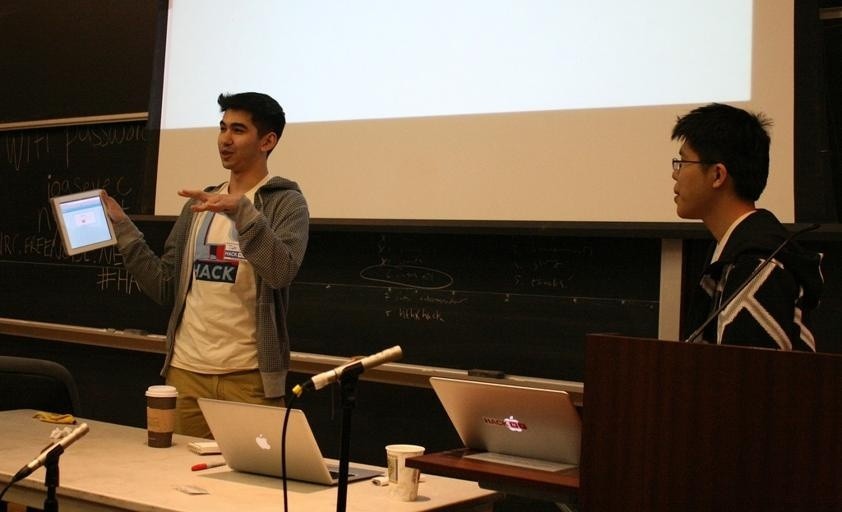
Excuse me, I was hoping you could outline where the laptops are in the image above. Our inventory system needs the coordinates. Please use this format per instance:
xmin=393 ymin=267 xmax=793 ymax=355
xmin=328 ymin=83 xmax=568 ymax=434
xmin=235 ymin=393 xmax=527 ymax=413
xmin=197 ymin=397 xmax=385 ymax=487
xmin=429 ymin=376 xmax=582 ymax=465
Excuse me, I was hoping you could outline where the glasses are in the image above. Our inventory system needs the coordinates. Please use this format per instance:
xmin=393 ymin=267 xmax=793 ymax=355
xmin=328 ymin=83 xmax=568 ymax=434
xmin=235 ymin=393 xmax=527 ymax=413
xmin=671 ymin=158 xmax=704 ymax=170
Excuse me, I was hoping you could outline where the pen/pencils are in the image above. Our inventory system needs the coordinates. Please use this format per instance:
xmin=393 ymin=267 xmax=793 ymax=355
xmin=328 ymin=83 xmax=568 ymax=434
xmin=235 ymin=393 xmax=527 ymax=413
xmin=192 ymin=461 xmax=227 ymax=471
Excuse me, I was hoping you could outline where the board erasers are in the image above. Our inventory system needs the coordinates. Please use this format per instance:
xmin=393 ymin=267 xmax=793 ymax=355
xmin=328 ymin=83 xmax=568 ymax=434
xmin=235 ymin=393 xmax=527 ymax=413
xmin=123 ymin=328 xmax=148 ymax=336
xmin=468 ymin=368 xmax=506 ymax=379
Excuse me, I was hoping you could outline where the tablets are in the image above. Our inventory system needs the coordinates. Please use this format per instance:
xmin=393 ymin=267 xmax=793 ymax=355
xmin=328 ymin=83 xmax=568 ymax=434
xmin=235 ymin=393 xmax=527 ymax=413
xmin=50 ymin=189 xmax=118 ymax=255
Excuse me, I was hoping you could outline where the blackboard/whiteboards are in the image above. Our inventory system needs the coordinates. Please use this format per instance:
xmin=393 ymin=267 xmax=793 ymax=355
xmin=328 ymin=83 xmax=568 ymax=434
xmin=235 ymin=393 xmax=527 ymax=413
xmin=0 ymin=0 xmax=842 ymax=405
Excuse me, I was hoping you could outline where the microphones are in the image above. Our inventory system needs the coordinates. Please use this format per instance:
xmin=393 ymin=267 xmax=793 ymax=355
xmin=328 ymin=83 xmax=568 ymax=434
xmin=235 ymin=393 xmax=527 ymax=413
xmin=14 ymin=423 xmax=90 ymax=479
xmin=291 ymin=345 xmax=403 ymax=399
xmin=683 ymin=221 xmax=821 ymax=343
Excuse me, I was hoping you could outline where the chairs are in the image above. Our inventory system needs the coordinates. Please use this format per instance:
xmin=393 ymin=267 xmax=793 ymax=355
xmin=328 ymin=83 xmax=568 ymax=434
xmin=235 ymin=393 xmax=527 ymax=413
xmin=283 ymin=369 xmax=348 ymax=458
xmin=1 ymin=353 xmax=80 ymax=415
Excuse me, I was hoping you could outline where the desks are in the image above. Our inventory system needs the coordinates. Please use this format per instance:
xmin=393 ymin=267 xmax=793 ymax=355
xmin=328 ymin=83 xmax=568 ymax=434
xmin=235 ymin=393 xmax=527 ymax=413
xmin=0 ymin=404 xmax=499 ymax=512
xmin=402 ymin=446 xmax=582 ymax=512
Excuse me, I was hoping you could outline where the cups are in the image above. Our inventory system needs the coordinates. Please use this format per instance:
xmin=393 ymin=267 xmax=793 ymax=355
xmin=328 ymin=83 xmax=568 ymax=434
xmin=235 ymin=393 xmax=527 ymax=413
xmin=385 ymin=442 xmax=425 ymax=501
xmin=145 ymin=385 xmax=179 ymax=448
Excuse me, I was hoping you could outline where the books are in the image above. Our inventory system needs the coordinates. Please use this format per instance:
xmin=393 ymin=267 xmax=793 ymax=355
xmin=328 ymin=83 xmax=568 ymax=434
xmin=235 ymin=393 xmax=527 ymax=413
xmin=186 ymin=439 xmax=222 ymax=454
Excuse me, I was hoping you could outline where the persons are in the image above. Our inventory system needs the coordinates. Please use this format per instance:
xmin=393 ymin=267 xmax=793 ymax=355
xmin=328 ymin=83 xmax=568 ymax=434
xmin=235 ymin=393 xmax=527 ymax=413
xmin=99 ymin=91 xmax=310 ymax=440
xmin=670 ymin=102 xmax=821 ymax=357
